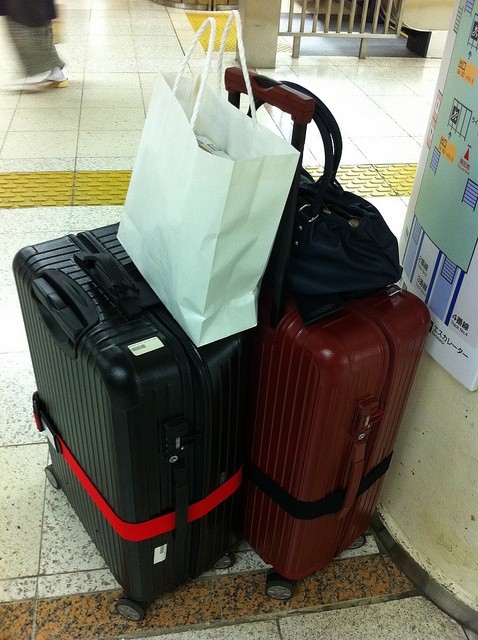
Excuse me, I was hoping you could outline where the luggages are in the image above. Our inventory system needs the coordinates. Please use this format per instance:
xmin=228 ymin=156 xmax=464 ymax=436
xmin=13 ymin=222 xmax=248 ymax=622
xmin=242 ymin=284 xmax=431 ymax=600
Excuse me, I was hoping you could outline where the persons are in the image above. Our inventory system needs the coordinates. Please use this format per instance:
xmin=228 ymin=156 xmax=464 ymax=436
xmin=0 ymin=1 xmax=69 ymax=93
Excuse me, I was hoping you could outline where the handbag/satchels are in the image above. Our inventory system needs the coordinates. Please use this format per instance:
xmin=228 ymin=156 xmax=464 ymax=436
xmin=115 ymin=9 xmax=301 ymax=348
xmin=247 ymin=80 xmax=404 ymax=326
